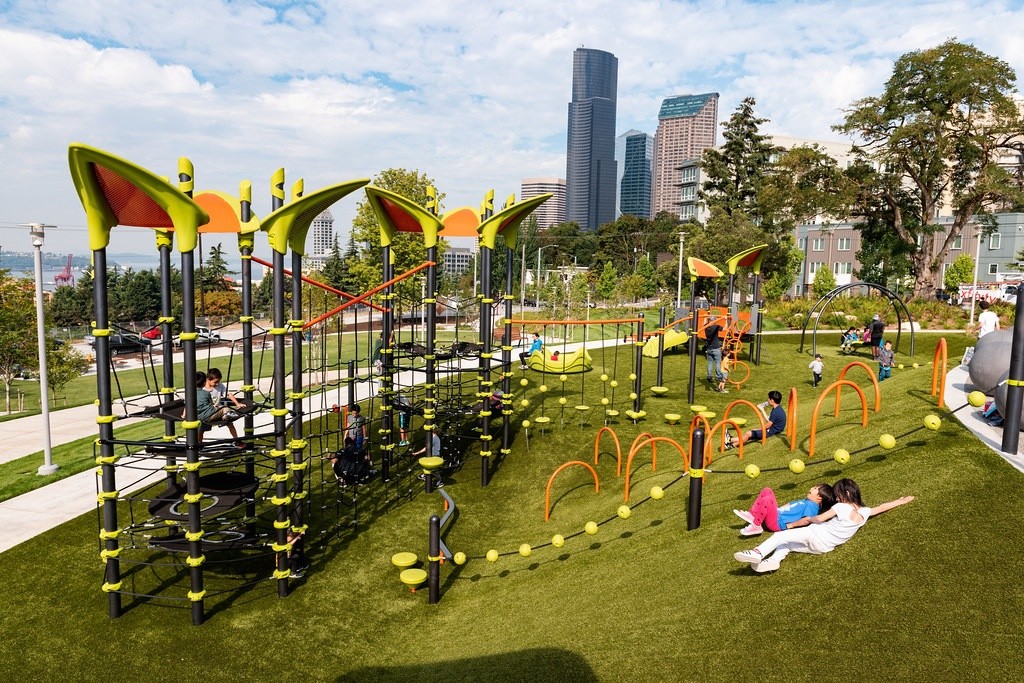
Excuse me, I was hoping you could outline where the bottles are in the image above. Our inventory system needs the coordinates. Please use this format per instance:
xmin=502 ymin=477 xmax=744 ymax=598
xmin=757 ymin=403 xmax=769 ymax=407
xmin=985 ymin=401 xmax=991 ymax=412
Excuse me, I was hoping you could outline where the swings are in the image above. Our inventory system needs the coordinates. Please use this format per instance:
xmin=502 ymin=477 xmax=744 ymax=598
xmin=830 ymin=300 xmax=894 ymax=348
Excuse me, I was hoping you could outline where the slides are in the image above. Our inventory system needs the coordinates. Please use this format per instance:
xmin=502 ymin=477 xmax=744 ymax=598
xmin=642 ymin=328 xmax=690 ymax=358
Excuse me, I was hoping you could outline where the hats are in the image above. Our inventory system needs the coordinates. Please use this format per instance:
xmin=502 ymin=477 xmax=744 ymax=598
xmin=815 ymin=354 xmax=823 ymax=358
xmin=873 ymin=315 xmax=880 ymax=321
xmin=491 ymin=390 xmax=504 ymax=401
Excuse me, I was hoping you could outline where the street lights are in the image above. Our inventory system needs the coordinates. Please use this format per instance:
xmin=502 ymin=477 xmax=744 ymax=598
xmin=471 ymin=252 xmax=477 ymax=298
xmin=676 ymin=231 xmax=690 ymax=308
xmin=24 ymin=222 xmax=59 ymax=476
xmin=967 ymin=222 xmax=984 ymax=326
xmin=535 ymin=244 xmax=559 ymax=314
xmin=561 ymin=252 xmax=578 ymax=273
xmin=419 ymin=276 xmax=428 ymax=347
xmin=633 ymin=246 xmax=649 ymax=309
xmin=585 ymin=291 xmax=592 ymax=340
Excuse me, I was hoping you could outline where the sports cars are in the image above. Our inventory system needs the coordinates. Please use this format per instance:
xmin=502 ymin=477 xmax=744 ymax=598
xmin=141 ymin=326 xmax=163 ymax=339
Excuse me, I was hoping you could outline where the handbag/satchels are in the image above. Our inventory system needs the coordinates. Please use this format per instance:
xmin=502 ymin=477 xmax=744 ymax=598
xmin=702 ymin=345 xmax=707 ymax=354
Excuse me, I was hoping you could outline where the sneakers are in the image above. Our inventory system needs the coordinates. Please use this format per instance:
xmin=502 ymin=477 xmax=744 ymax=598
xmin=732 ymin=509 xmax=755 ymax=524
xmin=725 ymin=428 xmax=731 ymax=444
xmin=518 ymin=364 xmax=528 ymax=370
xmin=433 ymin=482 xmax=444 ymax=488
xmin=232 ymin=440 xmax=247 ymax=448
xmin=399 ymin=441 xmax=410 ymax=446
xmin=715 ymin=377 xmax=722 ymax=380
xmin=740 ymin=522 xmax=763 ymax=535
xmin=198 ymin=430 xmax=203 ymax=443
xmin=418 ymin=474 xmax=426 ymax=481
xmin=725 ymin=443 xmax=735 ymax=449
xmin=716 ymin=388 xmax=729 ymax=393
xmin=707 ymin=376 xmax=713 ymax=382
xmin=751 ymin=557 xmax=780 ymax=572
xmin=733 ymin=549 xmax=762 ymax=564
xmin=222 ymin=411 xmax=239 ymax=420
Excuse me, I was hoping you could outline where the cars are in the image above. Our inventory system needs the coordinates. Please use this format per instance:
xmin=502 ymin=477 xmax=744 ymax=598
xmin=88 ymin=331 xmax=151 ymax=357
xmin=171 ymin=326 xmax=221 ymax=347
xmin=516 ymin=298 xmax=536 ymax=307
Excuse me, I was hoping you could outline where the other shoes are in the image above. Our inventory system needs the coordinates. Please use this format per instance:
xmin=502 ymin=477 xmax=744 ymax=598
xmin=870 ymin=356 xmax=878 ymax=360
xmin=852 ymin=351 xmax=859 ymax=355
xmin=813 ymin=384 xmax=819 ymax=388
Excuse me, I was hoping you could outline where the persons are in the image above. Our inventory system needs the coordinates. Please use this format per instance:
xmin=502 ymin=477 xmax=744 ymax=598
xmin=972 ymin=300 xmax=1001 ymax=341
xmin=518 ymin=332 xmax=560 ymax=370
xmin=809 ymin=354 xmax=824 ymax=388
xmin=840 ymin=315 xmax=895 ymax=383
xmin=724 ymin=390 xmax=786 ymax=451
xmin=181 ymin=368 xmax=246 ymax=449
xmin=325 ymin=392 xmax=444 ymax=489
xmin=703 ymin=316 xmax=730 ymax=394
xmin=733 ymin=479 xmax=916 ymax=573
